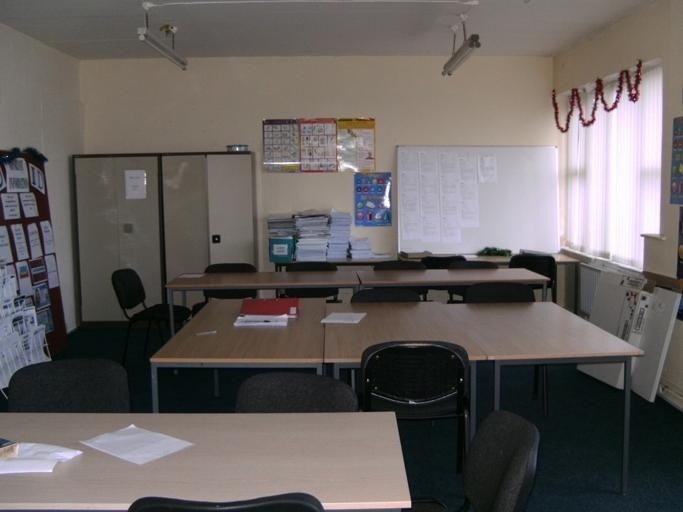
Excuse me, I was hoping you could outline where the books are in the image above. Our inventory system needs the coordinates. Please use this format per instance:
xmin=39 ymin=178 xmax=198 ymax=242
xmin=0 ymin=438 xmax=19 ymax=460
xmin=233 ymin=313 xmax=288 ymax=327
xmin=294 ymin=212 xmax=376 ymax=262
xmin=239 ymin=298 xmax=300 ymax=319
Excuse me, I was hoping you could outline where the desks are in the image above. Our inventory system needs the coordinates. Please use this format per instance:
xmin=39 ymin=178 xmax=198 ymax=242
xmin=1 ymin=408 xmax=412 ymax=509
xmin=323 ymin=301 xmax=646 ymax=500
xmin=149 ymin=297 xmax=325 ymax=408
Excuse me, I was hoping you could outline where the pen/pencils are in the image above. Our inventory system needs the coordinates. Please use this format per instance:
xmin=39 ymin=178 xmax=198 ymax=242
xmin=242 ymin=319 xmax=271 ymax=322
xmin=193 ymin=331 xmax=216 ymax=336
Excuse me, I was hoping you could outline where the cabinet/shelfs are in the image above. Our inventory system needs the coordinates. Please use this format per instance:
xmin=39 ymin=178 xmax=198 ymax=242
xmin=159 ymin=150 xmax=258 ymax=322
xmin=70 ymin=153 xmax=164 ymax=331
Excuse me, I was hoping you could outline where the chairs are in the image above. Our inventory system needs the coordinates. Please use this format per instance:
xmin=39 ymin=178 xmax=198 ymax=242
xmin=464 ymin=285 xmax=550 ymax=411
xmin=359 ymin=340 xmax=469 ymax=479
xmin=111 ymin=267 xmax=192 ymax=378
xmin=7 ymin=358 xmax=131 ymax=411
xmin=127 ymin=489 xmax=327 ymax=512
xmin=414 ymin=417 xmax=540 ymax=511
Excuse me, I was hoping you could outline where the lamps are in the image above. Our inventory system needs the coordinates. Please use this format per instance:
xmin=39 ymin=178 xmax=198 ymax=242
xmin=442 ymin=15 xmax=481 ymax=78
xmin=136 ymin=2 xmax=187 ymax=72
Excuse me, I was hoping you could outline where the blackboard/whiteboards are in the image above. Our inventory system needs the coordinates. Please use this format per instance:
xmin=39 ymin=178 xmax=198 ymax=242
xmin=397 ymin=145 xmax=561 ymax=256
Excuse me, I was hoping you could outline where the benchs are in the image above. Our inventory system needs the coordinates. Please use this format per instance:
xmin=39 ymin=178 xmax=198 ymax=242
xmin=165 ymin=256 xmax=564 ymax=340
